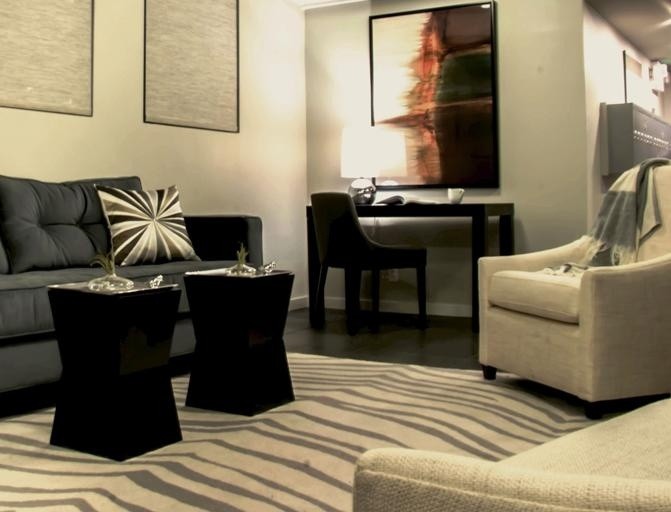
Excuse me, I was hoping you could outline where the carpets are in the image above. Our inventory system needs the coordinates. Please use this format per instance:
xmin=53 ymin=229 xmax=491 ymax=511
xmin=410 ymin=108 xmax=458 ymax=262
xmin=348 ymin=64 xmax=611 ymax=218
xmin=0 ymin=351 xmax=596 ymax=511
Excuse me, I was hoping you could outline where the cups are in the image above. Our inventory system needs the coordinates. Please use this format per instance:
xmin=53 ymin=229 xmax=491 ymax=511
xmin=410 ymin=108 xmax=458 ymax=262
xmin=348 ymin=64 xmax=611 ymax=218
xmin=448 ymin=188 xmax=464 ymax=204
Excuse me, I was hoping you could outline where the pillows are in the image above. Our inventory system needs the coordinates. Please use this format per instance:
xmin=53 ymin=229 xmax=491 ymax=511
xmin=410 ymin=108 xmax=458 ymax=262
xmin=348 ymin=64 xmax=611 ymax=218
xmin=93 ymin=182 xmax=202 ymax=265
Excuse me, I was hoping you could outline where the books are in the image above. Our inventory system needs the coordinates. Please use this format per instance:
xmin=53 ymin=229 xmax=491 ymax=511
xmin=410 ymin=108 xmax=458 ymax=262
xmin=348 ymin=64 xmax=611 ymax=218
xmin=377 ymin=193 xmax=442 ymax=206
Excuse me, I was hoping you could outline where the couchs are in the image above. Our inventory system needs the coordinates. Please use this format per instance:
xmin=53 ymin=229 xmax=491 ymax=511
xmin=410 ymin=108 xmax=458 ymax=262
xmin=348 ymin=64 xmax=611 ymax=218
xmin=0 ymin=176 xmax=263 ymax=394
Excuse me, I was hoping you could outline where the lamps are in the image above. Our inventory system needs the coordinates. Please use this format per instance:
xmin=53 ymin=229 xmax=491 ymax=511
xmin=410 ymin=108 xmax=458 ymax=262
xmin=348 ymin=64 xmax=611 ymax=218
xmin=340 ymin=125 xmax=406 ymax=204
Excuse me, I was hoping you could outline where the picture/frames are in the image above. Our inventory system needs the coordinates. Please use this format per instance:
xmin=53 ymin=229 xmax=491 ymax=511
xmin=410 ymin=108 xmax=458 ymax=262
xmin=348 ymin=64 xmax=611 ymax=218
xmin=368 ymin=0 xmax=500 ymax=189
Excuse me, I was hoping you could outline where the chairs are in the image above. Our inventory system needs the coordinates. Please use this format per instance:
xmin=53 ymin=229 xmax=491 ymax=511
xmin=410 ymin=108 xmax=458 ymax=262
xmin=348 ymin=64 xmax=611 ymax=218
xmin=310 ymin=191 xmax=429 ymax=330
xmin=354 ymin=392 xmax=671 ymax=512
xmin=477 ymin=165 xmax=671 ymax=418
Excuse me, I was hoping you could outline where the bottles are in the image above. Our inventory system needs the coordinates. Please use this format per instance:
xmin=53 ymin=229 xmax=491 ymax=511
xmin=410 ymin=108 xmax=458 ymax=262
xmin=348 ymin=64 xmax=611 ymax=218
xmin=348 ymin=177 xmax=376 ymax=204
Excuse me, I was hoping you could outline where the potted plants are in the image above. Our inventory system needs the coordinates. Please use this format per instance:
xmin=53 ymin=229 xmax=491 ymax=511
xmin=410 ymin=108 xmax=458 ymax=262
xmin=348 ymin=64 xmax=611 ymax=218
xmin=86 ymin=250 xmax=134 ymax=291
xmin=227 ymin=245 xmax=256 ymax=276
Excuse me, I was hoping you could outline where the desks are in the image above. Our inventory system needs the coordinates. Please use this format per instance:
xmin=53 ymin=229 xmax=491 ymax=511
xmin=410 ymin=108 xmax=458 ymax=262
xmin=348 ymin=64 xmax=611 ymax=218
xmin=306 ymin=204 xmax=515 ymax=331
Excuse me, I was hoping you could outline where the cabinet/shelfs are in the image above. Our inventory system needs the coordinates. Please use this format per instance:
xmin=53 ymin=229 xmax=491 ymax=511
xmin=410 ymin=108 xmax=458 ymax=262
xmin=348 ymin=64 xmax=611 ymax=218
xmin=607 ymin=103 xmax=671 ymax=175
